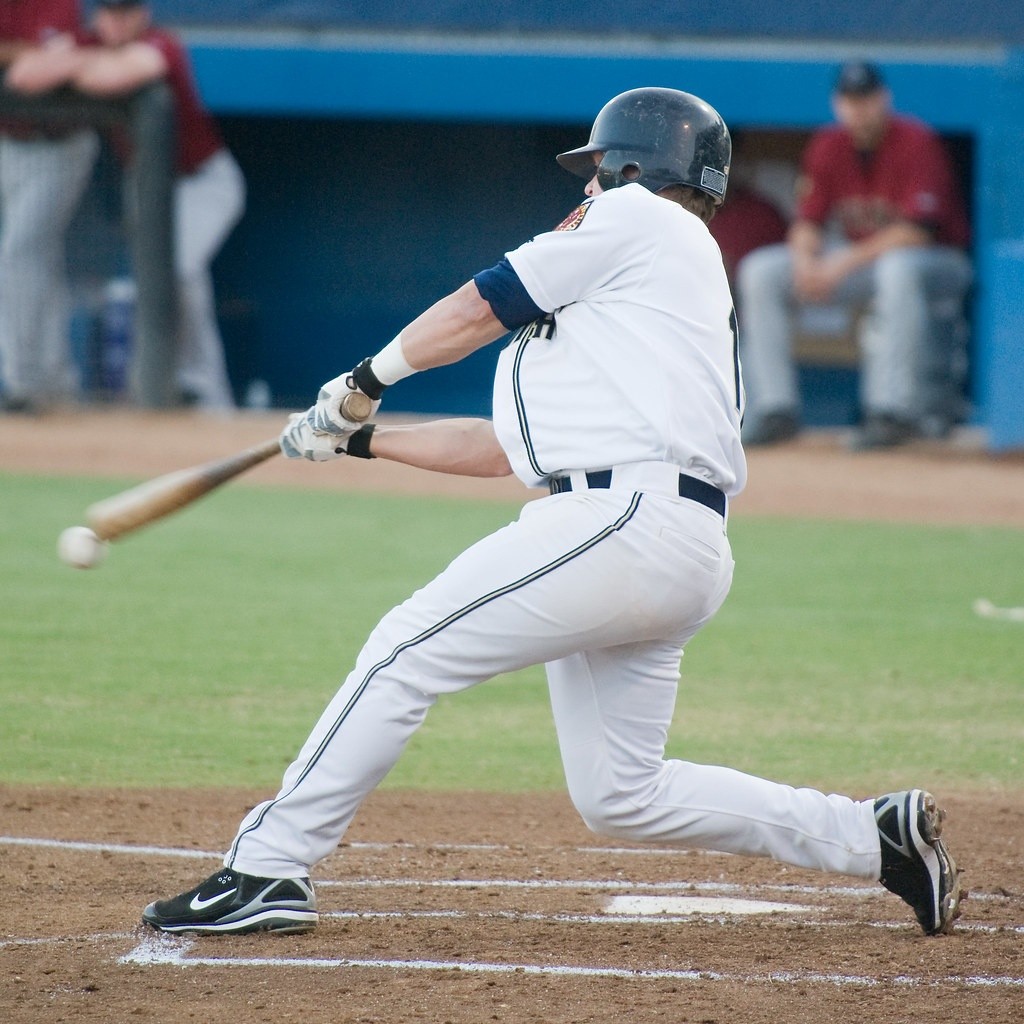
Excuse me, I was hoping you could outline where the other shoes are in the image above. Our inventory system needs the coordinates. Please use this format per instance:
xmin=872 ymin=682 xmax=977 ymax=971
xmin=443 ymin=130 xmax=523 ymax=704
xmin=742 ymin=414 xmax=799 ymax=448
xmin=861 ymin=415 xmax=910 ymax=452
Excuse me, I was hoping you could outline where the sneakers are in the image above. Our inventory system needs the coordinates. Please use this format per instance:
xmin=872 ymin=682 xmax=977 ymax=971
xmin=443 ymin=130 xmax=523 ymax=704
xmin=141 ymin=861 xmax=319 ymax=936
xmin=875 ymin=788 xmax=969 ymax=936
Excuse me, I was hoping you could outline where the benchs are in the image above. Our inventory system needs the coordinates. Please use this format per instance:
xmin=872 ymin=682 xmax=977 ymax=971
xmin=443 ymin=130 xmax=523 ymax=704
xmin=724 ymin=128 xmax=975 ymax=428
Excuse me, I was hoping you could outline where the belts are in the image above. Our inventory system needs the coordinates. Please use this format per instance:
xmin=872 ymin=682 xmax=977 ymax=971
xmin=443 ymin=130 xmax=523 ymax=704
xmin=549 ymin=467 xmax=725 ymax=516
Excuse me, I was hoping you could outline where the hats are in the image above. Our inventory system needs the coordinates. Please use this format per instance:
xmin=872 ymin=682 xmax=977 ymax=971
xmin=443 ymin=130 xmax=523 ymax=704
xmin=835 ymin=61 xmax=886 ymax=93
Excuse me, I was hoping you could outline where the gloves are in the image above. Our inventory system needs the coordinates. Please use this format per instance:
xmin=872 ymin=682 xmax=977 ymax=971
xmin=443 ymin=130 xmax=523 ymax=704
xmin=313 ymin=357 xmax=391 ymax=437
xmin=279 ymin=412 xmax=376 ymax=462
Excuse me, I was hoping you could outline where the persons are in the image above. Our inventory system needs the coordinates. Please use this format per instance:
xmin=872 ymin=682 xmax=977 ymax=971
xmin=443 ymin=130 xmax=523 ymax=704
xmin=139 ymin=86 xmax=959 ymax=936
xmin=738 ymin=65 xmax=973 ymax=455
xmin=0 ymin=0 xmax=250 ymax=409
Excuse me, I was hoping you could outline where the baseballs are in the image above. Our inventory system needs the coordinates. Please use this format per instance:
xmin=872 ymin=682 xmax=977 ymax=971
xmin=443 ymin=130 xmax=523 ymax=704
xmin=55 ymin=527 xmax=100 ymax=571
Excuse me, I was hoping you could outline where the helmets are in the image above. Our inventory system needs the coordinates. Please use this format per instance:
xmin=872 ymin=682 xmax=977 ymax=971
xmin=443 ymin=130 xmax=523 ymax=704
xmin=554 ymin=87 xmax=733 ymax=207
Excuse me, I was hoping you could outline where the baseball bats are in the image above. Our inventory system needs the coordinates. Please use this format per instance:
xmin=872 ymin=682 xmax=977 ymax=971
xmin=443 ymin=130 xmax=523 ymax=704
xmin=86 ymin=392 xmax=379 ymax=551
